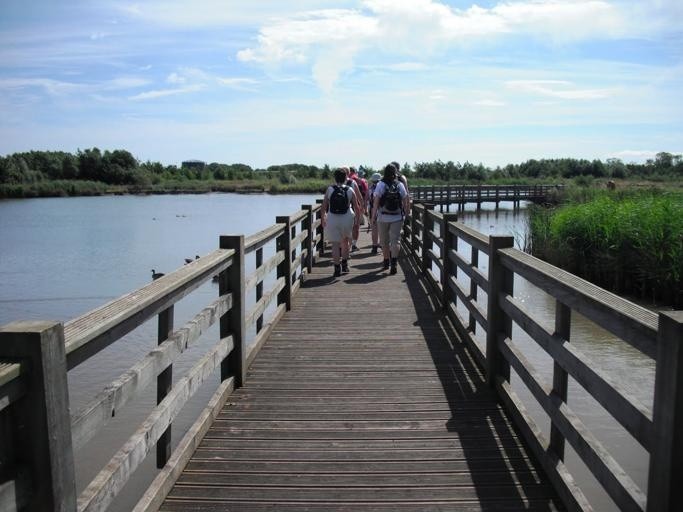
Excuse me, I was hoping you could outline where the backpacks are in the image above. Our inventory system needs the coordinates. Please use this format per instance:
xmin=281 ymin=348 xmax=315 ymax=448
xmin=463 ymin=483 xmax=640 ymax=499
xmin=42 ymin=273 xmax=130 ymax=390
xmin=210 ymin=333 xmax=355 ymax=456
xmin=329 ymin=184 xmax=352 ymax=215
xmin=356 ymin=178 xmax=366 ymax=197
xmin=378 ymin=178 xmax=403 ymax=216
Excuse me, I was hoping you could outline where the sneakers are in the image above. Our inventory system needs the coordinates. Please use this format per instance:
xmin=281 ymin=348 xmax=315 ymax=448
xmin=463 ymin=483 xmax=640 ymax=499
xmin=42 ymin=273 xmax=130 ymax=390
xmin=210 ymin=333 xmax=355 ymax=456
xmin=371 ymin=244 xmax=379 ymax=254
xmin=352 ymin=244 xmax=360 ymax=252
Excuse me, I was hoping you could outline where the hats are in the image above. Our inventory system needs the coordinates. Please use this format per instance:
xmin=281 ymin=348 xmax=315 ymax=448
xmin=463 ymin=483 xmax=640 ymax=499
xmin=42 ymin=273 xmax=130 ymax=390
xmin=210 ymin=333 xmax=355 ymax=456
xmin=369 ymin=172 xmax=383 ymax=181
xmin=358 ymin=170 xmax=367 ymax=177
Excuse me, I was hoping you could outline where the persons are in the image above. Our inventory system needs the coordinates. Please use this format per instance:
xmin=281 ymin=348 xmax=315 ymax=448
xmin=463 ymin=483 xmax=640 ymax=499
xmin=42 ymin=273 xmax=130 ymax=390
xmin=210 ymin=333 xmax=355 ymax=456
xmin=320 ymin=161 xmax=411 ymax=277
xmin=607 ymin=181 xmax=616 ymax=191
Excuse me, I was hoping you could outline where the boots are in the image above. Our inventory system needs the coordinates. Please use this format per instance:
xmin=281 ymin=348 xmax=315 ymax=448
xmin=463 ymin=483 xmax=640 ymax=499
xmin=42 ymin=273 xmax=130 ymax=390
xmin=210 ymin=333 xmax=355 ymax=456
xmin=390 ymin=257 xmax=398 ymax=275
xmin=341 ymin=259 xmax=350 ymax=273
xmin=334 ymin=263 xmax=341 ymax=277
xmin=383 ymin=257 xmax=390 ymax=270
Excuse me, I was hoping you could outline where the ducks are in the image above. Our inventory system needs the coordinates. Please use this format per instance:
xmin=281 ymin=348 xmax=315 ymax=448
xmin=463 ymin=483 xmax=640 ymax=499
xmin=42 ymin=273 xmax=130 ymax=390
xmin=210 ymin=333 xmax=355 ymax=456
xmin=150 ymin=269 xmax=164 ymax=277
xmin=183 ymin=255 xmax=199 ymax=263
